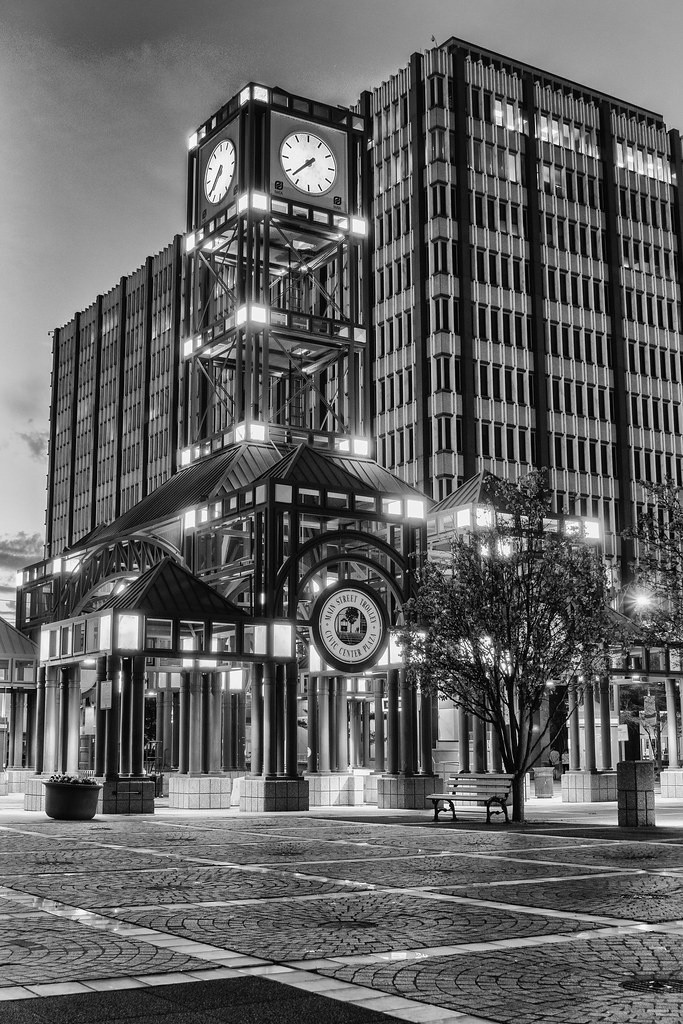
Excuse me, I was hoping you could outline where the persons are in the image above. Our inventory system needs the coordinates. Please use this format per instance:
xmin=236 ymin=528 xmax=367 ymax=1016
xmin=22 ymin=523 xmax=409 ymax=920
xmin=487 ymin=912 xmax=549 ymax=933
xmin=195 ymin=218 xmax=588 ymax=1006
xmin=561 ymin=749 xmax=569 ymax=774
xmin=550 ymin=746 xmax=560 ymax=780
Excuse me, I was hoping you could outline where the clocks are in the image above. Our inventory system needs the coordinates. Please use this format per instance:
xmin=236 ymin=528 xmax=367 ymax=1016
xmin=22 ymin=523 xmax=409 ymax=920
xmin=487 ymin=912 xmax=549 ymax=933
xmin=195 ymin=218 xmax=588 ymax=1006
xmin=278 ymin=131 xmax=338 ymax=198
xmin=203 ymin=139 xmax=237 ymax=206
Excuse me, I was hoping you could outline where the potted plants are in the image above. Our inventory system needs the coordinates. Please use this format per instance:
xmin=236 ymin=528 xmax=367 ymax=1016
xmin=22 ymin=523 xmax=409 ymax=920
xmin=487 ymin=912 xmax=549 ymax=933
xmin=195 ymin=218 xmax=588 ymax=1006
xmin=40 ymin=774 xmax=104 ymax=821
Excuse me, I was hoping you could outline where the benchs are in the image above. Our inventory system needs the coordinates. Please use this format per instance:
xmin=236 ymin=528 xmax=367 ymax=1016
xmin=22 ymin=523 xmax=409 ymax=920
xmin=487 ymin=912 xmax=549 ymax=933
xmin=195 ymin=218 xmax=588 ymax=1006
xmin=426 ymin=769 xmax=519 ymax=823
